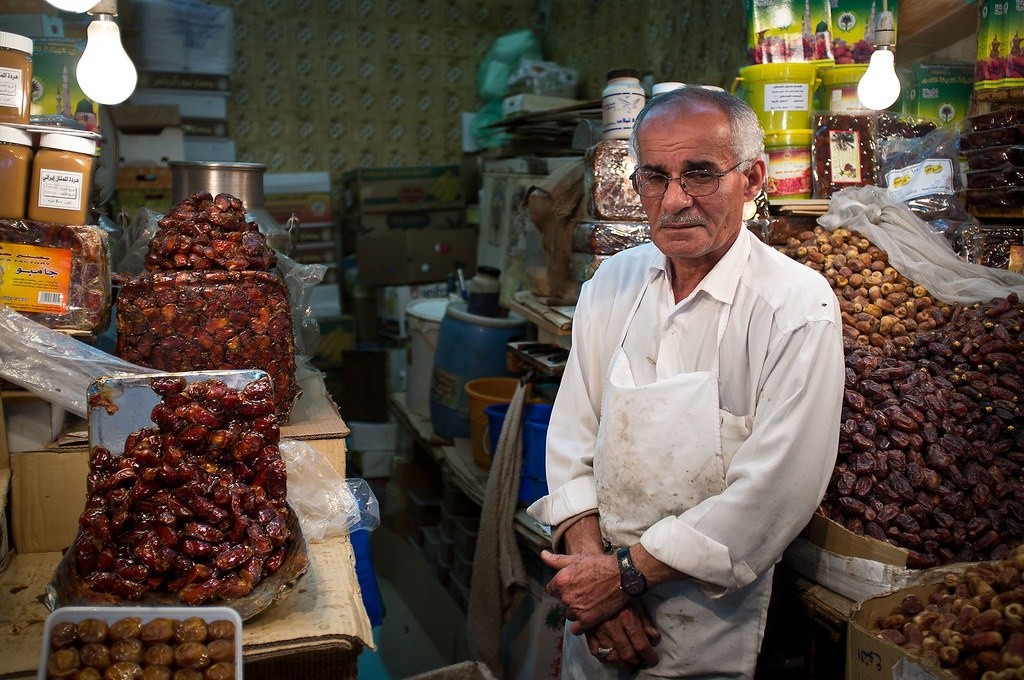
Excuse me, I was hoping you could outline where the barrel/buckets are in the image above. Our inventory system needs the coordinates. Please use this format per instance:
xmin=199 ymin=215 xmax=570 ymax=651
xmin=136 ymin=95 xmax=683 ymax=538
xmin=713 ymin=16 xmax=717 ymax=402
xmin=466 ymin=377 xmax=546 ymax=471
xmin=430 ymin=300 xmax=531 ymax=438
xmin=730 ymin=62 xmax=821 ymax=130
xmin=763 ymin=129 xmax=815 ymax=199
xmin=821 ymin=63 xmax=896 ymax=120
xmin=485 ymin=403 xmax=554 ymax=504
xmin=406 ymin=298 xmax=449 ymax=418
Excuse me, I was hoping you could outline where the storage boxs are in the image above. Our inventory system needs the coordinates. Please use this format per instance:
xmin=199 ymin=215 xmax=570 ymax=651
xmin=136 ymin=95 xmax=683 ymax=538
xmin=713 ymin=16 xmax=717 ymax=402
xmin=503 ymin=576 xmax=566 ymax=680
xmin=116 ymin=188 xmax=173 ymax=215
xmin=280 ymin=219 xmax=344 ymax=287
xmin=783 ymin=511 xmax=1024 ymax=603
xmin=354 ymin=208 xmax=475 ymax=286
xmin=846 ymin=582 xmax=964 ymax=680
xmin=262 ymin=171 xmax=333 ymax=225
xmin=476 ymin=171 xmax=551 ymax=271
xmin=30 ymin=38 xmax=101 ymax=135
xmin=309 ymin=283 xmax=342 ymax=318
xmin=117 ymin=164 xmax=173 ymax=190
xmin=502 ymin=93 xmax=578 ymax=120
xmin=343 ymin=163 xmax=467 ymax=214
xmin=115 ymin=127 xmax=185 ymax=168
xmin=8 ymin=365 xmax=353 ymax=555
xmin=346 ymin=410 xmax=483 ymax=663
xmin=369 ymin=282 xmax=447 ymax=342
xmin=900 ymin=63 xmax=974 ymax=135
xmin=744 ymin=0 xmax=899 ymax=66
xmin=351 ymin=347 xmax=408 ymax=395
xmin=131 ymin=1 xmax=234 ymax=76
xmin=109 ymin=103 xmax=180 ymax=130
xmin=37 ymin=607 xmax=245 ymax=680
xmin=974 ymin=0 xmax=1024 ymax=94
xmin=124 ymin=73 xmax=230 ymax=137
xmin=316 ymin=314 xmax=358 ymax=369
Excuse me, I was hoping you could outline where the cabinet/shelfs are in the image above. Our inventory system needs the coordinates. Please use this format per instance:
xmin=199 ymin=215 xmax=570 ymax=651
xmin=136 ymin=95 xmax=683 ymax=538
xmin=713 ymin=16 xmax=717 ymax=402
xmin=0 ymin=329 xmax=98 ymax=577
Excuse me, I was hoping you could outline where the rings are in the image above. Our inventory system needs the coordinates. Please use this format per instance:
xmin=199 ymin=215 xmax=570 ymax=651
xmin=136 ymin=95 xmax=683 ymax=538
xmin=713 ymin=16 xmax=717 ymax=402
xmin=596 ymin=647 xmax=617 ymax=658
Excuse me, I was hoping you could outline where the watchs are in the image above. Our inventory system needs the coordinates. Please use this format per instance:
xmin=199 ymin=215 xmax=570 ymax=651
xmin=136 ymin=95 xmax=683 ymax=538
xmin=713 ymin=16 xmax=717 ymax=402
xmin=616 ymin=545 xmax=648 ymax=598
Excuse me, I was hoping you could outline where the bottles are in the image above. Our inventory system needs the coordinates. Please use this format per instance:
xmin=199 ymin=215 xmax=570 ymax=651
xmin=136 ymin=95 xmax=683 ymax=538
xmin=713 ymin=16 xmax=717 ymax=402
xmin=467 ymin=265 xmax=501 ymax=316
xmin=603 ymin=70 xmax=646 ymax=140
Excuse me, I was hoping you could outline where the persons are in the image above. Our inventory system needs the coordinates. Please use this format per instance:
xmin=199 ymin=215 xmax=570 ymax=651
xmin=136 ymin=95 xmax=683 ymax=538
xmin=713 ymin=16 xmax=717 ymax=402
xmin=522 ymin=86 xmax=848 ymax=680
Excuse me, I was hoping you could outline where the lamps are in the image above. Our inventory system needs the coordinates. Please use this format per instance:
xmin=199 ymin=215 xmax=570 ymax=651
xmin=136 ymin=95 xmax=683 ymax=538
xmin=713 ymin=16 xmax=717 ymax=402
xmin=858 ymin=0 xmax=901 ymax=112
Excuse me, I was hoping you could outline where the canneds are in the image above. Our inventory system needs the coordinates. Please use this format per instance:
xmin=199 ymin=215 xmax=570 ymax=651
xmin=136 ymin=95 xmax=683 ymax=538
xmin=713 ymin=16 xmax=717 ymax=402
xmin=26 ymin=132 xmax=97 ymax=227
xmin=0 ymin=31 xmax=34 ymax=123
xmin=0 ymin=125 xmax=34 ymax=219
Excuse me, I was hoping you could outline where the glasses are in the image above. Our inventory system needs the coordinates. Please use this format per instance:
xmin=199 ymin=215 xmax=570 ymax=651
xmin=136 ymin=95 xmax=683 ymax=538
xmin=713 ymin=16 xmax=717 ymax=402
xmin=629 ymin=160 xmax=753 ymax=197
